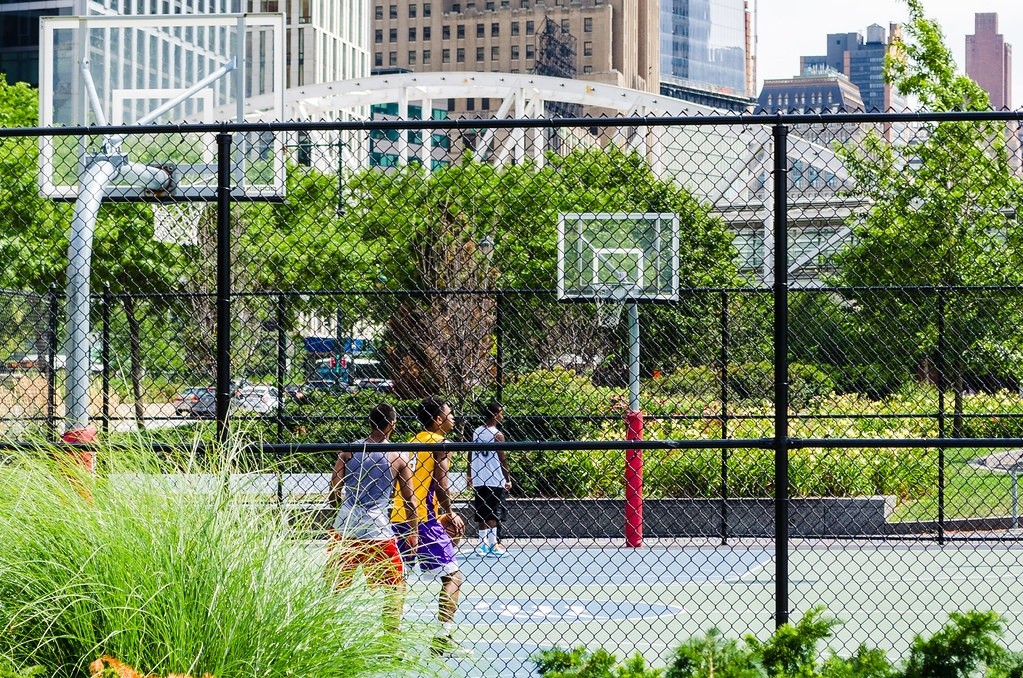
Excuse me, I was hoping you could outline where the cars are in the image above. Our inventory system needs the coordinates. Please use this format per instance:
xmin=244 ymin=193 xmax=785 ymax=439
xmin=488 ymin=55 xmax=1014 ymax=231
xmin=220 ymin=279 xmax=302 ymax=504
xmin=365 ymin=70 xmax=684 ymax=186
xmin=193 ymin=388 xmax=217 ymax=417
xmin=175 ymin=388 xmax=197 ymax=415
xmin=234 ymin=387 xmax=279 ymax=413
xmin=283 ymin=380 xmax=393 ymax=401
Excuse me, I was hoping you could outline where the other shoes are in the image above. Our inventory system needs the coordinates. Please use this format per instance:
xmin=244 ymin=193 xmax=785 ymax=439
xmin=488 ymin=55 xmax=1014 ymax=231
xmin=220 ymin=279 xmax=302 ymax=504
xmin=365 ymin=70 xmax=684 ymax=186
xmin=477 ymin=548 xmax=510 ymax=558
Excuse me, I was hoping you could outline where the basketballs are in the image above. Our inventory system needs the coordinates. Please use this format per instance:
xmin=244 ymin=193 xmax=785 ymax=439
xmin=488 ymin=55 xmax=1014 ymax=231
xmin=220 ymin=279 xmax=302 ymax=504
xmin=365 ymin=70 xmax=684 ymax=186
xmin=440 ymin=514 xmax=464 ymax=549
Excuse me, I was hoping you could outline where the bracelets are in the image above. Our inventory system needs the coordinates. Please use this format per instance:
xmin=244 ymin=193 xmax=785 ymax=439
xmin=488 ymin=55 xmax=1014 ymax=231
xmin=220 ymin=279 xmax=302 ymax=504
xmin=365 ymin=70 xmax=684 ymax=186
xmin=506 ymin=481 xmax=511 ymax=485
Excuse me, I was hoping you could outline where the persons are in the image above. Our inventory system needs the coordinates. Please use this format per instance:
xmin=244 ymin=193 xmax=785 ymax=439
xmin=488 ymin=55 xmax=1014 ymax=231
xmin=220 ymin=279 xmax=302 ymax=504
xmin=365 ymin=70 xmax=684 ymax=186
xmin=391 ymin=397 xmax=473 ymax=658
xmin=323 ymin=403 xmax=418 ymax=634
xmin=466 ymin=403 xmax=512 ymax=558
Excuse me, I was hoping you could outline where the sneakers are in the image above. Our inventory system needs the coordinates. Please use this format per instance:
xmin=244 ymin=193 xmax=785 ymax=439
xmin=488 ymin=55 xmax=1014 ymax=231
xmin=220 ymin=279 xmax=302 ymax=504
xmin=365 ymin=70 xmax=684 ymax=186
xmin=430 ymin=638 xmax=469 ymax=658
